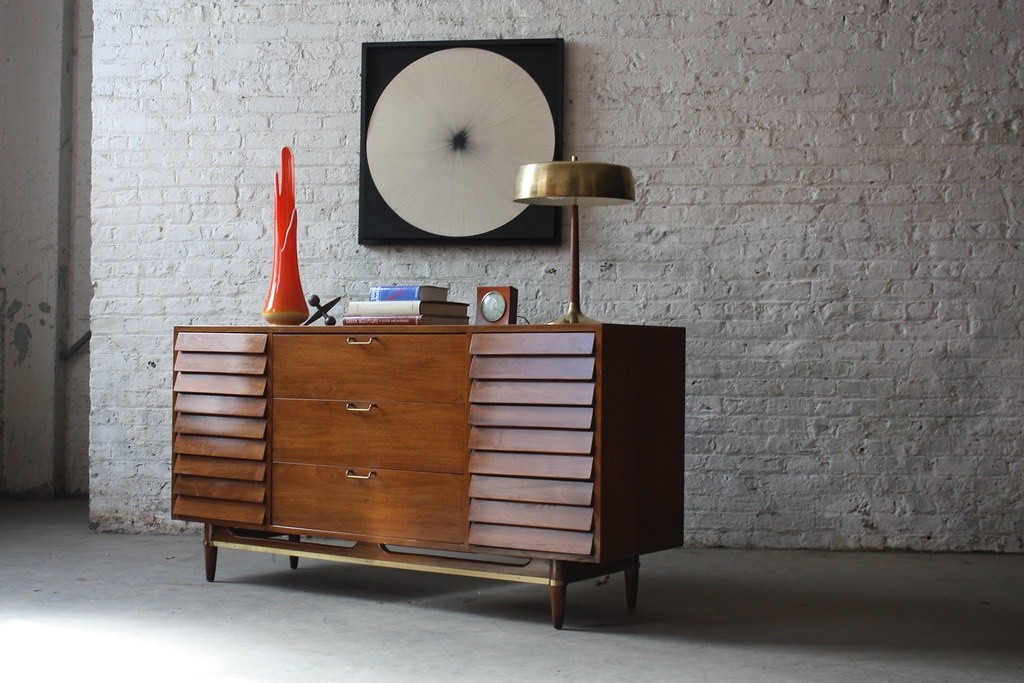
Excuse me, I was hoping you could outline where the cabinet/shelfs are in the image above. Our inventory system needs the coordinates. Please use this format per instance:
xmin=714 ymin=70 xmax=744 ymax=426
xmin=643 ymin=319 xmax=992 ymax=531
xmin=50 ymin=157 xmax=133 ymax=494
xmin=168 ymin=323 xmax=687 ymax=629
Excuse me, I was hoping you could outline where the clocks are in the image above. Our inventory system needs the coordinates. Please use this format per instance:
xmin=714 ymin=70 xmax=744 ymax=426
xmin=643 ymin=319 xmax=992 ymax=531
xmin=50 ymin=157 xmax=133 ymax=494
xmin=473 ymin=285 xmax=517 ymax=324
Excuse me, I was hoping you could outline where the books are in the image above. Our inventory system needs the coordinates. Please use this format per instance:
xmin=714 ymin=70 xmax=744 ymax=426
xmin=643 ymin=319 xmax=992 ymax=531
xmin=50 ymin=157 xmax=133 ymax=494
xmin=342 ymin=284 xmax=470 ymax=330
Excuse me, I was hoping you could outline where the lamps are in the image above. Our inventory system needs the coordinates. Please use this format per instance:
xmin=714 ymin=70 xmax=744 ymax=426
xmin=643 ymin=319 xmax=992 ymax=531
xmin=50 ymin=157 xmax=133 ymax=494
xmin=512 ymin=154 xmax=637 ymax=325
